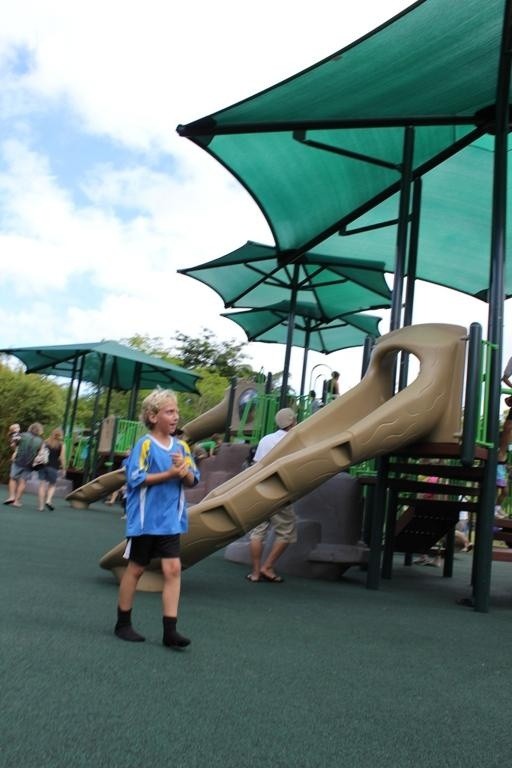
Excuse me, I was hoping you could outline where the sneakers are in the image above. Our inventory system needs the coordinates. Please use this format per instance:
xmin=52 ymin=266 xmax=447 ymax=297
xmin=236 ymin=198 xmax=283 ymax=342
xmin=494 ymin=506 xmax=509 ymax=520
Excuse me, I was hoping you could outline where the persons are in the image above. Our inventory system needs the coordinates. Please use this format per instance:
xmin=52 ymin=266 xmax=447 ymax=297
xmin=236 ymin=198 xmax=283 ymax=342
xmin=4 ymin=423 xmax=66 ymax=512
xmin=310 ymin=390 xmax=325 ymax=414
xmin=326 ymin=371 xmax=340 ymax=401
xmin=105 ymin=384 xmax=201 ymax=647
xmin=493 ymin=430 xmax=509 ymax=519
xmin=193 ymin=433 xmax=222 ymax=462
xmin=244 ymin=407 xmax=297 ymax=583
xmin=413 ymin=459 xmax=474 ymax=567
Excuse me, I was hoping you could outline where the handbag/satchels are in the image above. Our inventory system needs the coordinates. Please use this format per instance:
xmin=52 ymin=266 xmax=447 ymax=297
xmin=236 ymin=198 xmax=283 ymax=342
xmin=32 ymin=441 xmax=51 ymax=470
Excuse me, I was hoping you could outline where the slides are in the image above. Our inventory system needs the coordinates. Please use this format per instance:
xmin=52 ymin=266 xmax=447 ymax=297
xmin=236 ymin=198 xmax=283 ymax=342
xmin=65 ymin=383 xmax=261 ymax=510
xmin=99 ymin=324 xmax=467 ymax=591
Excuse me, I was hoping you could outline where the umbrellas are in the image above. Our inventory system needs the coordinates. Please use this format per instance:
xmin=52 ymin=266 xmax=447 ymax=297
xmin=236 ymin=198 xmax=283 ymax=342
xmin=176 ymin=241 xmax=393 ymax=354
xmin=178 ymin=0 xmax=512 ymax=300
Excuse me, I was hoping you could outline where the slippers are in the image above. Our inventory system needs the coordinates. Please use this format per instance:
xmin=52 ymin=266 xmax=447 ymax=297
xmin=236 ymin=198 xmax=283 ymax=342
xmin=3 ymin=496 xmax=55 ymax=512
xmin=413 ymin=559 xmax=440 ymax=568
xmin=462 ymin=543 xmax=473 ymax=554
xmin=245 ymin=573 xmax=260 ymax=583
xmin=260 ymin=569 xmax=283 ymax=582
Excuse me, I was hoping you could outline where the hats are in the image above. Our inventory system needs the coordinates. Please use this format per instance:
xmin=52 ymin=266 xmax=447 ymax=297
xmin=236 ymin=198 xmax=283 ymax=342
xmin=275 ymin=406 xmax=299 ymax=429
